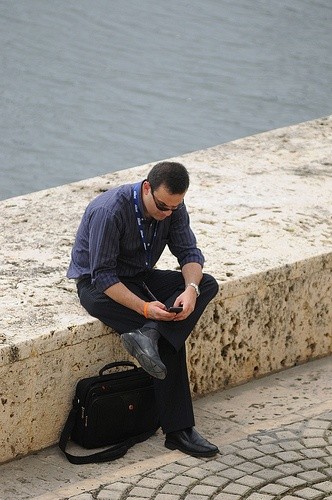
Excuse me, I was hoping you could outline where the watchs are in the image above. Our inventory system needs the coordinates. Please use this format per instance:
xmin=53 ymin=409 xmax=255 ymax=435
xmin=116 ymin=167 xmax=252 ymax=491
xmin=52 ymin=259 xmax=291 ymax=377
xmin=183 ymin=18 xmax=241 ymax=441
xmin=185 ymin=283 xmax=200 ymax=297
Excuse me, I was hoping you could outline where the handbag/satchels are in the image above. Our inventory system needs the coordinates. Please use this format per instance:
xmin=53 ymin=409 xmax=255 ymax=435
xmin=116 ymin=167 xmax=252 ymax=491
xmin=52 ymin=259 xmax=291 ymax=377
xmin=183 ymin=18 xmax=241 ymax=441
xmin=58 ymin=360 xmax=160 ymax=465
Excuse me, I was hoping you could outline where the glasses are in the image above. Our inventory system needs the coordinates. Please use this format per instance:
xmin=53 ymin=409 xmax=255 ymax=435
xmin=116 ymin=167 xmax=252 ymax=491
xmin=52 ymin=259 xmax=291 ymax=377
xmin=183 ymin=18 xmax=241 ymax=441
xmin=150 ymin=184 xmax=184 ymax=212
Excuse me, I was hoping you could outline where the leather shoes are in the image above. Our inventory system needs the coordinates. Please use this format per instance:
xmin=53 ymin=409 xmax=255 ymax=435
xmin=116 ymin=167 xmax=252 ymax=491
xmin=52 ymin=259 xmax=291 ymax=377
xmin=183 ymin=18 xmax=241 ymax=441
xmin=120 ymin=329 xmax=166 ymax=379
xmin=164 ymin=426 xmax=219 ymax=457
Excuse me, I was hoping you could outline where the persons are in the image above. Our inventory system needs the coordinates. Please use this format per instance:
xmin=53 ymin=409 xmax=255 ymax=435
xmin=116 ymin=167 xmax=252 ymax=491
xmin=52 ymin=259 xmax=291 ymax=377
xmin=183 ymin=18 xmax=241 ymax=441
xmin=66 ymin=162 xmax=219 ymax=457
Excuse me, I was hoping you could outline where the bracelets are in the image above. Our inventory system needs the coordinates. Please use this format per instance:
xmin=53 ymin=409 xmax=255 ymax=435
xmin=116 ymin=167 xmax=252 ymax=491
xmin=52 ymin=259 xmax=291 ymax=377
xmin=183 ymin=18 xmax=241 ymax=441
xmin=144 ymin=302 xmax=149 ymax=318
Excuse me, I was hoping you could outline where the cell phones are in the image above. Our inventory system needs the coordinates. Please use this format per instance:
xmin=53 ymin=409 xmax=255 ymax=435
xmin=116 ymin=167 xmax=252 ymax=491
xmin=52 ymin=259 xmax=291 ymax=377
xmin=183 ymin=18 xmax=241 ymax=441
xmin=166 ymin=307 xmax=183 ymax=313
